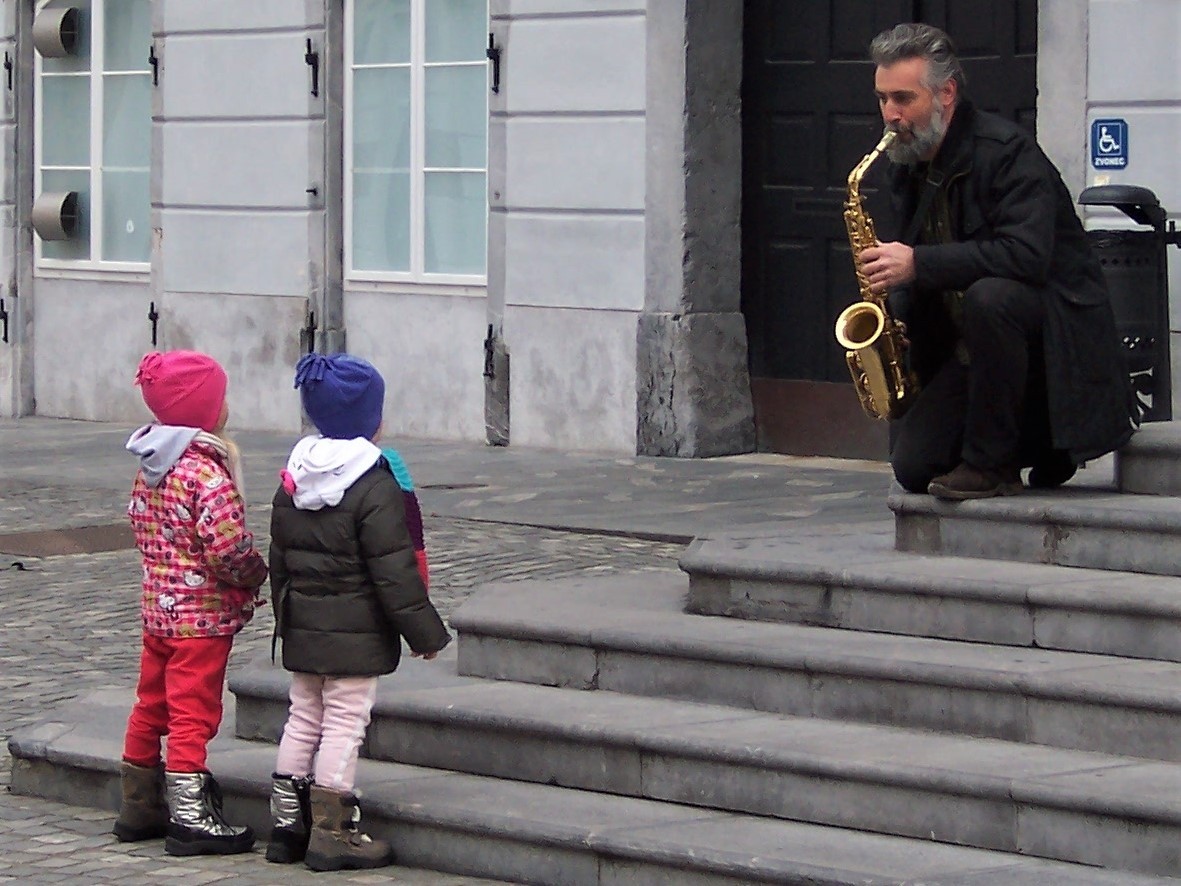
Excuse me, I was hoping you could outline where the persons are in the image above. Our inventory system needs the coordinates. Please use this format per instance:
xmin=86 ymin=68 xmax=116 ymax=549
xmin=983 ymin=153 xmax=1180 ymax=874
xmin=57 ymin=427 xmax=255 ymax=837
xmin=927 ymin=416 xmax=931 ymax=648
xmin=111 ymin=350 xmax=269 ymax=855
xmin=860 ymin=23 xmax=1141 ymax=498
xmin=265 ymin=353 xmax=453 ymax=875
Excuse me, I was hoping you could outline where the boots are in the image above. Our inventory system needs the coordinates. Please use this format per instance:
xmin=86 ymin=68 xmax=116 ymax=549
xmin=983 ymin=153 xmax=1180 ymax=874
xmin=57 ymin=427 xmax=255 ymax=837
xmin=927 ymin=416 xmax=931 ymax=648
xmin=161 ymin=769 xmax=256 ymax=857
xmin=112 ymin=759 xmax=173 ymax=842
xmin=264 ymin=773 xmax=315 ymax=865
xmin=302 ymin=785 xmax=394 ymax=873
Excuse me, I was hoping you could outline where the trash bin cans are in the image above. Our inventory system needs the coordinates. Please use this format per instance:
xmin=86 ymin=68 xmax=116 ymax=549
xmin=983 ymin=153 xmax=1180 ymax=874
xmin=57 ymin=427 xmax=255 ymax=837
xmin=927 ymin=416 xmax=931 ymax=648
xmin=1078 ymin=184 xmax=1181 ymax=422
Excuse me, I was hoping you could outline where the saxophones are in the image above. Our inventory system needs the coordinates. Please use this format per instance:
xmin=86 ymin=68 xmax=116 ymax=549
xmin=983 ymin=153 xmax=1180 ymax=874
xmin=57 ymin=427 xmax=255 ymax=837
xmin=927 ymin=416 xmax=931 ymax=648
xmin=835 ymin=130 xmax=913 ymax=421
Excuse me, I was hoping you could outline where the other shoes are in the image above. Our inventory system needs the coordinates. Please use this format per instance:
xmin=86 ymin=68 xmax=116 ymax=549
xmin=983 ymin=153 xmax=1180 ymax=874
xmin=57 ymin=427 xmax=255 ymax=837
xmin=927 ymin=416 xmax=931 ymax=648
xmin=927 ymin=462 xmax=1025 ymax=501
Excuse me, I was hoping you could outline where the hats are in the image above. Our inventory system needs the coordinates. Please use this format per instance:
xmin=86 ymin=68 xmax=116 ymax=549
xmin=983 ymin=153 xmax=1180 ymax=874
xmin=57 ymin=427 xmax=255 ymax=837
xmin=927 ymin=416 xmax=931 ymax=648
xmin=134 ymin=351 xmax=227 ymax=430
xmin=294 ymin=350 xmax=386 ymax=442
